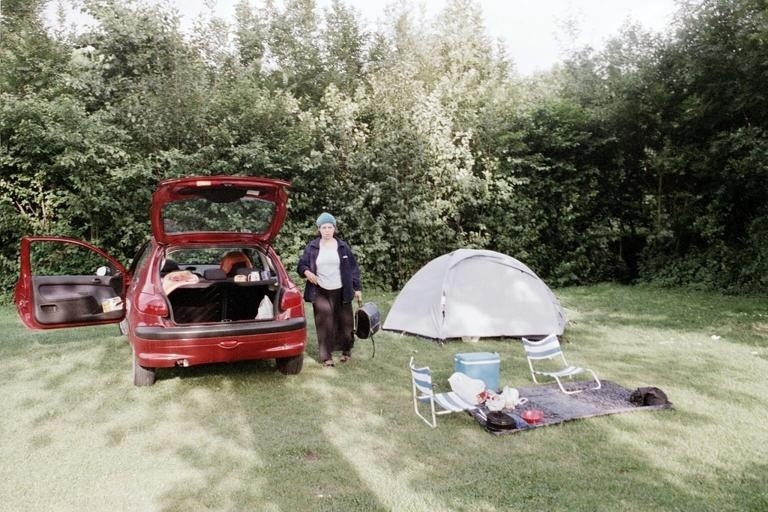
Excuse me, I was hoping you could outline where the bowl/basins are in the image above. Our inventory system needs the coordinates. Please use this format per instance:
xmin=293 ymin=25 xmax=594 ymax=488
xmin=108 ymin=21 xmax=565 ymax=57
xmin=522 ymin=410 xmax=543 ymax=423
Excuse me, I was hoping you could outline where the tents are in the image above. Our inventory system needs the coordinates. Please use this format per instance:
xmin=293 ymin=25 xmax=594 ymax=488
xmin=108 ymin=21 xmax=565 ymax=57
xmin=382 ymin=249 xmax=568 ymax=339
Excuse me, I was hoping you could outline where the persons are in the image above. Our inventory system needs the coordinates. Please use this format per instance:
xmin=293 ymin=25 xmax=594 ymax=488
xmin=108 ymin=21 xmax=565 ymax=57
xmin=297 ymin=212 xmax=362 ymax=367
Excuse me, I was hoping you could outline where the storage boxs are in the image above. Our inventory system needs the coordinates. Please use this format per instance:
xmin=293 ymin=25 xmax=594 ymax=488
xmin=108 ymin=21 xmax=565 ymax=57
xmin=454 ymin=352 xmax=500 ymax=390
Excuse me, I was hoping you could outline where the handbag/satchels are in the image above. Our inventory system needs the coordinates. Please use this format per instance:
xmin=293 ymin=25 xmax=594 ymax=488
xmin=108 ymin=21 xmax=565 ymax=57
xmin=630 ymin=387 xmax=668 ymax=405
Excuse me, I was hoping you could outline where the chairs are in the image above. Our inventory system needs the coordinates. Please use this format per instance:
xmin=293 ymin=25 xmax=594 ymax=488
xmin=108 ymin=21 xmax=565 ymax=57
xmin=522 ymin=333 xmax=601 ymax=394
xmin=409 ymin=356 xmax=487 ymax=428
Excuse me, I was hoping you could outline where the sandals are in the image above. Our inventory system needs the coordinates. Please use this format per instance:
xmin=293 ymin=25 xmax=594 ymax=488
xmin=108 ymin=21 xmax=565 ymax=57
xmin=324 ymin=352 xmax=351 ymax=368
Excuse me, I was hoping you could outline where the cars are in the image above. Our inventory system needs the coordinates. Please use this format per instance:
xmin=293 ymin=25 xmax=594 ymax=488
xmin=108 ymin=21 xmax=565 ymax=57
xmin=10 ymin=172 xmax=307 ymax=390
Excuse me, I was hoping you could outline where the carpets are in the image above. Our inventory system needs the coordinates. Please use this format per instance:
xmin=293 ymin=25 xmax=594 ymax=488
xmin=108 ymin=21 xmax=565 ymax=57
xmin=466 ymin=381 xmax=665 ymax=436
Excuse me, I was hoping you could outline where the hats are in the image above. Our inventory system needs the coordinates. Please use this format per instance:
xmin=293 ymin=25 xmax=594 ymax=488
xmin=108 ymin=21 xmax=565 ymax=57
xmin=316 ymin=212 xmax=336 ymax=226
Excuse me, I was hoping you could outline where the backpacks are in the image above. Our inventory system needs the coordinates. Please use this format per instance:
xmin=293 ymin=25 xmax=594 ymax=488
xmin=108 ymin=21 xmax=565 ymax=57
xmin=354 ymin=302 xmax=380 ymax=340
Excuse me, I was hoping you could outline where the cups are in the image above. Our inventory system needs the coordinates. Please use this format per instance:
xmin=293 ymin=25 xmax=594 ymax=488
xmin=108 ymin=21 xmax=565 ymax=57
xmin=249 ymin=271 xmax=270 ymax=282
